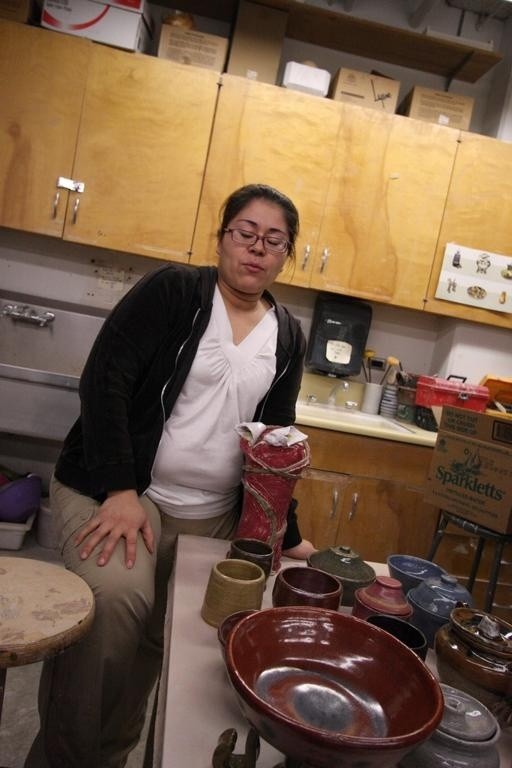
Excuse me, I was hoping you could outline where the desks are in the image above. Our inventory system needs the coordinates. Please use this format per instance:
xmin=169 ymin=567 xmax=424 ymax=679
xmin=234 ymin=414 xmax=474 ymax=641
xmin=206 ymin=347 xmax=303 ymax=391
xmin=144 ymin=536 xmax=512 ymax=768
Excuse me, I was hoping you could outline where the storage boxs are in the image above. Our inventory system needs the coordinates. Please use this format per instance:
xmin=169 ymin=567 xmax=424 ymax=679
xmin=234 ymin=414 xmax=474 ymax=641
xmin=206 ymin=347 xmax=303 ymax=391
xmin=42 ymin=0 xmax=150 ymax=52
xmin=332 ymin=67 xmax=400 ymax=115
xmin=434 ymin=405 xmax=512 ymax=536
xmin=403 ymin=85 xmax=474 ymax=131
xmin=158 ymin=22 xmax=229 ymax=73
xmin=98 ymin=0 xmax=155 ymax=37
xmin=0 ymin=512 xmax=37 ymax=550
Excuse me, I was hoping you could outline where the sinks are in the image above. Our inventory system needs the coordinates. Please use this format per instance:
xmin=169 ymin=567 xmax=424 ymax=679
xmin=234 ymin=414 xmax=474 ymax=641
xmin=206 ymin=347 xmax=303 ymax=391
xmin=296 ymin=401 xmax=412 ymax=435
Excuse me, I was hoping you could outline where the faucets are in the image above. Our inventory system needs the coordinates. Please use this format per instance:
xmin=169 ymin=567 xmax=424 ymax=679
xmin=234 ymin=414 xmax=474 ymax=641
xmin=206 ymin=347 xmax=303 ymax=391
xmin=328 ymin=380 xmax=349 ymax=405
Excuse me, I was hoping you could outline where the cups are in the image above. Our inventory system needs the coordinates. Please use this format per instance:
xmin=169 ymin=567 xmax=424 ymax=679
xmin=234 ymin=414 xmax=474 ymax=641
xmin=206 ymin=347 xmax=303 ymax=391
xmin=362 ymin=382 xmax=383 ymax=414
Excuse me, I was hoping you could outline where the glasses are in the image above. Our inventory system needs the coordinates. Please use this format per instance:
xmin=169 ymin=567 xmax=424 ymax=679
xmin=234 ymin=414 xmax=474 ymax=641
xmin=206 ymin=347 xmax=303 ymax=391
xmin=222 ymin=227 xmax=291 ymax=254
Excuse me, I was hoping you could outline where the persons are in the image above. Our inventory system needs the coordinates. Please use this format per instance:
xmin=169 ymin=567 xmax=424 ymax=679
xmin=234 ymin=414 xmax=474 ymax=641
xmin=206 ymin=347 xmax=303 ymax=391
xmin=25 ymin=184 xmax=319 ymax=768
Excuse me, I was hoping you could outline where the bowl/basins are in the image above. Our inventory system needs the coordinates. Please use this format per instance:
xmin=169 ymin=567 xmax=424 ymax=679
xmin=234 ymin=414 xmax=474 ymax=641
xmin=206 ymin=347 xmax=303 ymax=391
xmin=272 ymin=565 xmax=344 ymax=612
xmin=386 ymin=554 xmax=447 ymax=589
xmin=362 ymin=614 xmax=428 ymax=662
xmin=228 ymin=605 xmax=444 ymax=767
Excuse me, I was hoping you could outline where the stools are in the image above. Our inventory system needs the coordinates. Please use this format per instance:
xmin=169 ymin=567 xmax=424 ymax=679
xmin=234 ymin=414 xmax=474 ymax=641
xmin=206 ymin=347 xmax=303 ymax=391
xmin=1 ymin=555 xmax=95 ymax=768
xmin=428 ymin=510 xmax=510 ymax=624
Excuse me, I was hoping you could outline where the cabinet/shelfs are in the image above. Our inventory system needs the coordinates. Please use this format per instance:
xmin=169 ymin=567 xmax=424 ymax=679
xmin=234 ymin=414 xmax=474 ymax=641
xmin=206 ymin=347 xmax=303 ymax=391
xmin=293 ymin=402 xmax=436 ymax=566
xmin=188 ymin=75 xmax=462 ymax=314
xmin=423 ymin=131 xmax=512 ymax=331
xmin=1 ymin=20 xmax=222 ymax=264
xmin=272 ymin=1 xmax=512 ymax=143
